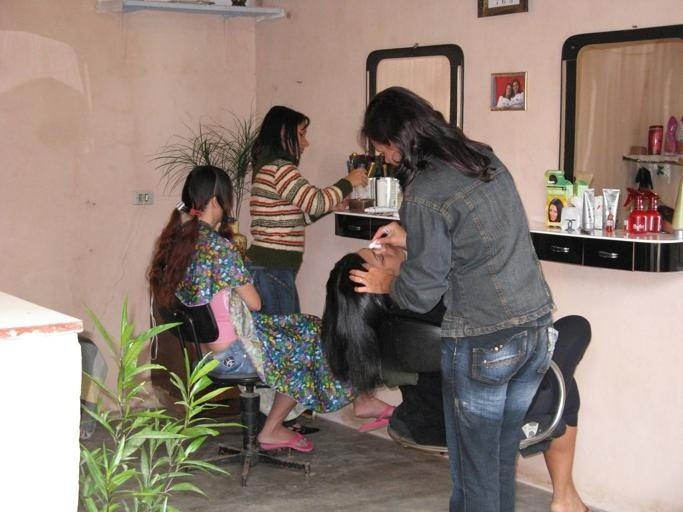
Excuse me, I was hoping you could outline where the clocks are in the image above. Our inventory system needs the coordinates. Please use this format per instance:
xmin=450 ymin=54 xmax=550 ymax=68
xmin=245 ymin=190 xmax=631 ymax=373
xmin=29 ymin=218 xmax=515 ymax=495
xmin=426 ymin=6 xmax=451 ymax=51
xmin=477 ymin=0 xmax=528 ymax=17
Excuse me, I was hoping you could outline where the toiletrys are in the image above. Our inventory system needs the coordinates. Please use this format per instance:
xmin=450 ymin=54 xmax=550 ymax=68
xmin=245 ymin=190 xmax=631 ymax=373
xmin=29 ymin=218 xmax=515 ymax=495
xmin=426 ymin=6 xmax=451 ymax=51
xmin=562 ymin=185 xmax=620 ymax=234
xmin=646 ymin=124 xmax=662 ymax=155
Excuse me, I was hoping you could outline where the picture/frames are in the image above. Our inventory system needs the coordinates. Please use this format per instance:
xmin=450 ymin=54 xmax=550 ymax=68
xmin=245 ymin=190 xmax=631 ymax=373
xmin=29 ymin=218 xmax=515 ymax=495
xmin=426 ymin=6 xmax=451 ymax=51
xmin=492 ymin=72 xmax=528 ymax=111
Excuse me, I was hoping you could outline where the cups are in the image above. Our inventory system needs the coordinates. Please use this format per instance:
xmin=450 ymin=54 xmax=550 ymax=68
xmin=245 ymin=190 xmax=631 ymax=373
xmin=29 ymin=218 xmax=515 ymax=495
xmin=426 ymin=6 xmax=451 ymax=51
xmin=648 ymin=125 xmax=663 ymax=155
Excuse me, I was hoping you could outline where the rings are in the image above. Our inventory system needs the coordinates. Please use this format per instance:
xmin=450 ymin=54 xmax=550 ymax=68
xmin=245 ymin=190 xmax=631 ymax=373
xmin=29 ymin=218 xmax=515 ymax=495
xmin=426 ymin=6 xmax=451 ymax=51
xmin=359 ymin=277 xmax=364 ymax=283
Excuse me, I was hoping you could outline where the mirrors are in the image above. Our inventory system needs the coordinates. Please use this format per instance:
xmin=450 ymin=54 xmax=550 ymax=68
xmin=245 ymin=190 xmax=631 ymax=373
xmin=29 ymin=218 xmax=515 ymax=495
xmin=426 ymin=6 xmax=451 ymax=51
xmin=561 ymin=24 xmax=683 ymax=233
xmin=365 ymin=43 xmax=462 ymax=159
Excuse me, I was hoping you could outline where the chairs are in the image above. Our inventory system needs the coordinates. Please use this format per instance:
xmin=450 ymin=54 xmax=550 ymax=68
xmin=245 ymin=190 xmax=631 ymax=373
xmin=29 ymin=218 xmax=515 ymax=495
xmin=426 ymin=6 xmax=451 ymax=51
xmin=386 ymin=317 xmax=569 ymax=459
xmin=153 ymin=294 xmax=313 ymax=489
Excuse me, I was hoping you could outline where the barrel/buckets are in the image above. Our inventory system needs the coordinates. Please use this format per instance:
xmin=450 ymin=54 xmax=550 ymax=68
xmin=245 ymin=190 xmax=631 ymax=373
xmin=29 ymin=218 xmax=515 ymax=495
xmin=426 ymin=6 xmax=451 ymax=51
xmin=376 ymin=175 xmax=400 ymax=211
xmin=351 ymin=178 xmax=375 ymax=199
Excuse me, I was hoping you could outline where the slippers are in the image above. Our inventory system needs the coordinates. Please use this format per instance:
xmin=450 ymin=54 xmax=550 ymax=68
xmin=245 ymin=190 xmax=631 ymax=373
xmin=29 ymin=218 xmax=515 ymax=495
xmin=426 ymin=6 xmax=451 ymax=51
xmin=356 ymin=404 xmax=399 ymax=435
xmin=258 ymin=430 xmax=314 ymax=454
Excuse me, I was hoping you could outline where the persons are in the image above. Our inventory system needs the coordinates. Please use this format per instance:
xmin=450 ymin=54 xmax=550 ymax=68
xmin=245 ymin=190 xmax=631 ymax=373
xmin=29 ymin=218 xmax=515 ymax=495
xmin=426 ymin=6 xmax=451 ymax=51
xmin=319 ymin=242 xmax=595 ymax=512
xmin=243 ymin=105 xmax=369 ymax=434
xmin=548 ymin=197 xmax=563 ymax=223
xmin=509 ymin=80 xmax=523 ymax=108
xmin=145 ymin=163 xmax=396 ymax=452
xmin=349 ymin=85 xmax=558 ymax=512
xmin=495 ymin=83 xmax=512 ymax=108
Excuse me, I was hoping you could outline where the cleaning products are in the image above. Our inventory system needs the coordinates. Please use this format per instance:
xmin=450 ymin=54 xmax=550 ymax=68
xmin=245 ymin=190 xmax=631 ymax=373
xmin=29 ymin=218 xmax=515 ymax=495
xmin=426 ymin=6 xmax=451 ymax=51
xmin=623 ymin=187 xmax=647 ymax=233
xmin=638 ymin=187 xmax=663 ymax=232
